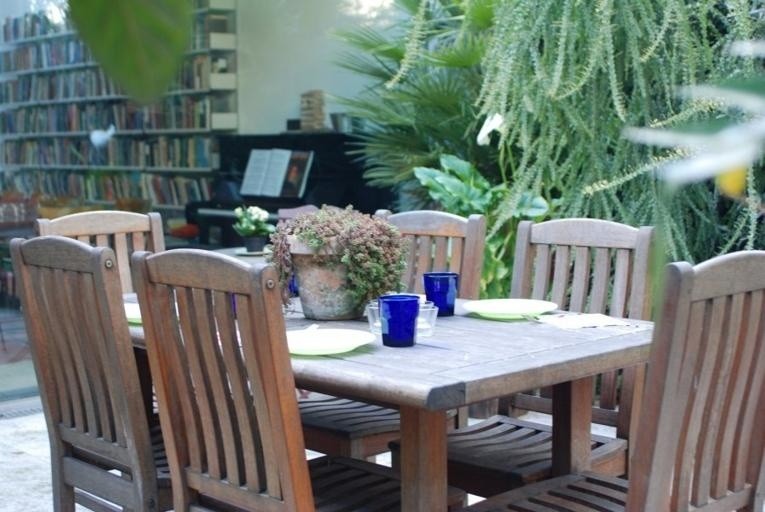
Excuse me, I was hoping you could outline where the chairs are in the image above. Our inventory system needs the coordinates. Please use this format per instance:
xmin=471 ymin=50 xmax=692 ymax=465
xmin=35 ymin=209 xmax=167 ymax=295
xmin=390 ymin=218 xmax=658 ymax=506
xmin=8 ymin=235 xmax=173 ymax=511
xmin=456 ymin=249 xmax=764 ymax=511
xmin=131 ymin=245 xmax=400 ymax=512
xmin=287 ymin=208 xmax=488 ymax=459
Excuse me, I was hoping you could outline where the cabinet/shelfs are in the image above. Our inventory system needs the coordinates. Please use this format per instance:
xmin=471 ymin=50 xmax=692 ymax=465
xmin=0 ymin=7 xmax=239 ymax=248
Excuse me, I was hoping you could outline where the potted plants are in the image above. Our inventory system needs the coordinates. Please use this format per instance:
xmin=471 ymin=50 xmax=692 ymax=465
xmin=268 ymin=204 xmax=408 ymax=326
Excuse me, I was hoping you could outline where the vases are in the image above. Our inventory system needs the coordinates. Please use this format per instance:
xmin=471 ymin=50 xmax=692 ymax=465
xmin=244 ymin=236 xmax=267 ymax=252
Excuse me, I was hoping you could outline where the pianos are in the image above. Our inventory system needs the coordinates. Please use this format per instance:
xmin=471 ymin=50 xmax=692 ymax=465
xmin=183 ymin=130 xmax=396 ymax=244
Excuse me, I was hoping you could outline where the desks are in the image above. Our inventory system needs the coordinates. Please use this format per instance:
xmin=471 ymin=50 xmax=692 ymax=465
xmin=121 ymin=295 xmax=657 ymax=512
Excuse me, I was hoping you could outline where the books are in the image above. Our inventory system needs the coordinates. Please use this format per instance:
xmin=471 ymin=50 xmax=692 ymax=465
xmin=240 ymin=146 xmax=315 ymax=203
xmin=1 ymin=6 xmax=236 ymax=229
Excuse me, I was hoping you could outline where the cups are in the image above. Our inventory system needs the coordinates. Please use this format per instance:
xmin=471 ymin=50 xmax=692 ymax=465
xmin=423 ymin=272 xmax=458 ymax=316
xmin=366 ymin=294 xmax=439 ymax=347
xmin=289 ymin=275 xmax=299 ymax=297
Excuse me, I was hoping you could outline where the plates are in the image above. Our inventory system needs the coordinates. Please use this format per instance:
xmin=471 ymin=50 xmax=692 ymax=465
xmin=286 ymin=329 xmax=375 ymax=355
xmin=462 ymin=299 xmax=558 ymax=319
xmin=124 ymin=303 xmax=180 ymax=323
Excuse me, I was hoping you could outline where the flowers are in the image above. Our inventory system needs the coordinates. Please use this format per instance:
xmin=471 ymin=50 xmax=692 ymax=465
xmin=232 ymin=203 xmax=277 ymax=237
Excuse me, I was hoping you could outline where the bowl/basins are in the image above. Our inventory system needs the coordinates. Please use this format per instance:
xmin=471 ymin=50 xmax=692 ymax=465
xmin=330 ymin=113 xmax=363 ymax=134
